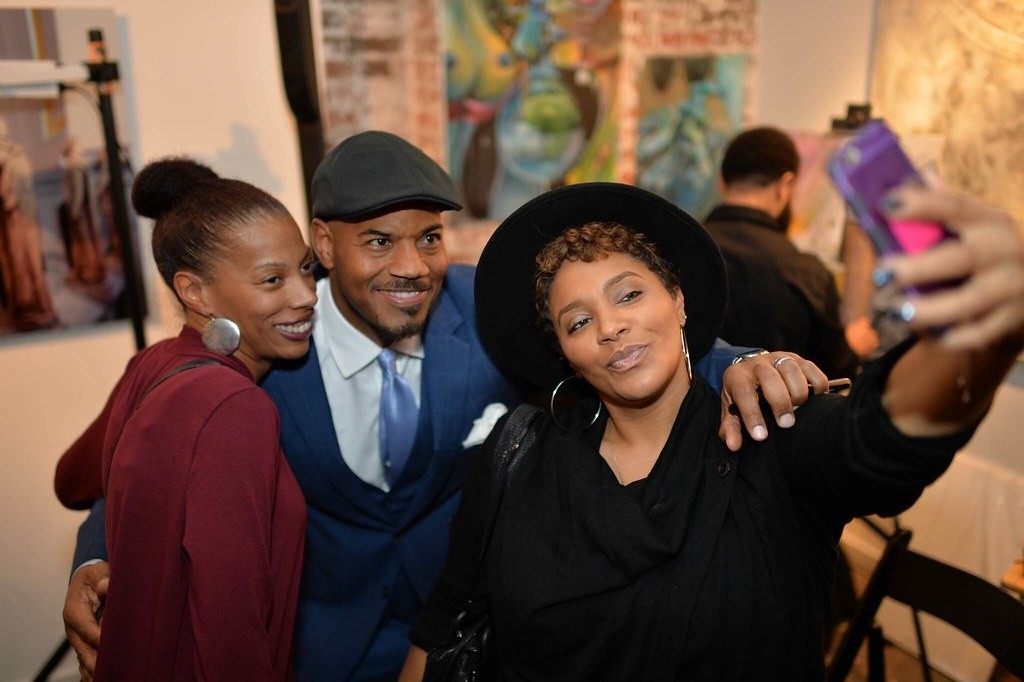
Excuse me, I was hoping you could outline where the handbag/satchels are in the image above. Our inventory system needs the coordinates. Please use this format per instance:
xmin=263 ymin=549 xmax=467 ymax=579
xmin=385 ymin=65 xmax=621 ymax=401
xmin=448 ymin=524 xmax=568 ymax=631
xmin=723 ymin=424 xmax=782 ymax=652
xmin=422 ymin=598 xmax=495 ymax=682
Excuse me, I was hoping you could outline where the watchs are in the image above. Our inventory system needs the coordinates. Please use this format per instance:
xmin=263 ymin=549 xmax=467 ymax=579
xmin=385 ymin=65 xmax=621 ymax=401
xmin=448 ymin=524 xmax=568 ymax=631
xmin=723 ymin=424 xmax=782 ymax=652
xmin=729 ymin=350 xmax=769 ymax=362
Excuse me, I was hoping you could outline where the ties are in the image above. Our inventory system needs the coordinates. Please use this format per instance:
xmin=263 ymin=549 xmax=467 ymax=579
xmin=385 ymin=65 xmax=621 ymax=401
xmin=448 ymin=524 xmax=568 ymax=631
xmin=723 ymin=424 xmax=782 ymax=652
xmin=378 ymin=348 xmax=418 ymax=491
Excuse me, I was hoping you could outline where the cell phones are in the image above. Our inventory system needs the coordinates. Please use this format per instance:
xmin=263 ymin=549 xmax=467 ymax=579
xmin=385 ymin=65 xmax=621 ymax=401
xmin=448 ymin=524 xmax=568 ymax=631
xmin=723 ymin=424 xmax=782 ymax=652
xmin=823 ymin=117 xmax=973 ymax=339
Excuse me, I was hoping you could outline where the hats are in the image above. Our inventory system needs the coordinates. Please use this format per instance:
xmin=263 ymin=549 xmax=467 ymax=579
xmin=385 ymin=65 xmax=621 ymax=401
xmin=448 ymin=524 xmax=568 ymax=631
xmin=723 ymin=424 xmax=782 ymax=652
xmin=475 ymin=181 xmax=728 ymax=400
xmin=310 ymin=131 xmax=462 ymax=224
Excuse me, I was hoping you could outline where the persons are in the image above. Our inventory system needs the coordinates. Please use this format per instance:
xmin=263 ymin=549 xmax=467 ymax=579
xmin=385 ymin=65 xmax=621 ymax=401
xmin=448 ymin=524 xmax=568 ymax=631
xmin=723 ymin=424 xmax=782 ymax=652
xmin=52 ymin=153 xmax=322 ymax=682
xmin=395 ymin=185 xmax=1023 ymax=682
xmin=66 ymin=129 xmax=834 ymax=682
xmin=700 ymin=128 xmax=856 ymax=386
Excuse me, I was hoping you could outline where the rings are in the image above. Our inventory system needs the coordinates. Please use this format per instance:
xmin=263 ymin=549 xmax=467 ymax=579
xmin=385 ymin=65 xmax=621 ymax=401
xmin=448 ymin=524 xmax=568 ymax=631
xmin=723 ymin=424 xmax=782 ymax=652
xmin=772 ymin=355 xmax=793 ymax=368
